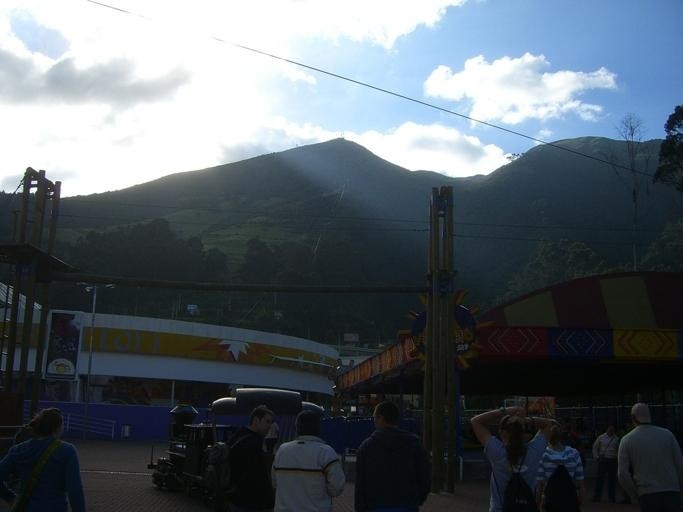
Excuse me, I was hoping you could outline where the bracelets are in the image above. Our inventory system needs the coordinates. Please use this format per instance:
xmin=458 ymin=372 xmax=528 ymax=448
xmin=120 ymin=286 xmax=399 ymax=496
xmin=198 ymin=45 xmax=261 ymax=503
xmin=498 ymin=406 xmax=505 ymax=414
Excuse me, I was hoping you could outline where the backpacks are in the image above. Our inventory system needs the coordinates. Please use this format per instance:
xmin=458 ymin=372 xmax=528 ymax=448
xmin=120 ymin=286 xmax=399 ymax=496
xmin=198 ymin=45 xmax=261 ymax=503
xmin=500 ymin=445 xmax=538 ymax=512
xmin=193 ymin=432 xmax=257 ymax=507
xmin=543 ymin=464 xmax=582 ymax=512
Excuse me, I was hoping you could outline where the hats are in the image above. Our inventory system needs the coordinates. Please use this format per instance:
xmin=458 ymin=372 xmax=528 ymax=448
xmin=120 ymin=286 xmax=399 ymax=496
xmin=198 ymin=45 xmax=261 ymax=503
xmin=631 ymin=403 xmax=651 ymax=424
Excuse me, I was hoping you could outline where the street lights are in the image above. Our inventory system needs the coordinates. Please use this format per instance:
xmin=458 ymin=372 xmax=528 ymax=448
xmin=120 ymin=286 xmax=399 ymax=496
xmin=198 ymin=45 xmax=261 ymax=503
xmin=75 ymin=281 xmax=117 ymax=441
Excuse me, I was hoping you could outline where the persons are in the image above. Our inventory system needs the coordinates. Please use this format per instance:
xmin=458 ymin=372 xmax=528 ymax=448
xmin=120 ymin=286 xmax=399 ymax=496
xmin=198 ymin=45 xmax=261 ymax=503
xmin=616 ymin=402 xmax=683 ymax=512
xmin=204 ymin=404 xmax=273 ymax=511
xmin=0 ymin=407 xmax=84 ymax=512
xmin=471 ymin=405 xmax=551 ymax=511
xmin=354 ymin=398 xmax=430 ymax=512
xmin=267 ymin=408 xmax=347 ymax=511
xmin=587 ymin=425 xmax=621 ymax=502
xmin=536 ymin=426 xmax=585 ymax=511
